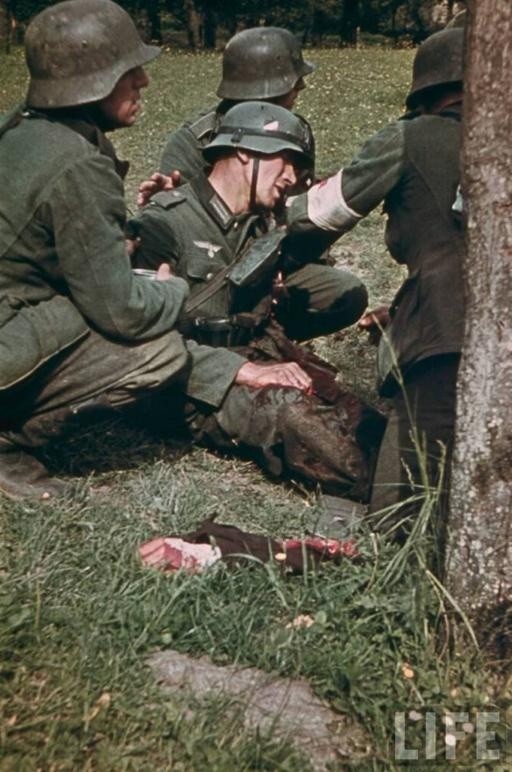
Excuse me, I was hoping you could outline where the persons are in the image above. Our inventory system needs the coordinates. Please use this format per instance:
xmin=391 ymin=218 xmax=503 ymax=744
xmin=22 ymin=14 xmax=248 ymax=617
xmin=275 ymin=26 xmax=468 ymax=549
xmin=134 ymin=514 xmax=370 ymax=588
xmin=131 ymin=24 xmax=370 ymax=348
xmin=1 ymin=2 xmax=193 ymax=507
xmin=124 ymin=99 xmax=387 ymax=506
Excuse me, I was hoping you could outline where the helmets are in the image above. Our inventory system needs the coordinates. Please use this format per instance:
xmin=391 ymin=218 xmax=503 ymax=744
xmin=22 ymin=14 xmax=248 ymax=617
xmin=201 ymin=99 xmax=318 ymax=171
xmin=21 ymin=1 xmax=165 ymax=111
xmin=405 ymin=24 xmax=468 ymax=109
xmin=212 ymin=25 xmax=319 ymax=107
